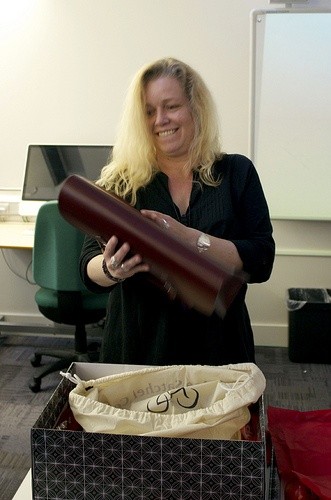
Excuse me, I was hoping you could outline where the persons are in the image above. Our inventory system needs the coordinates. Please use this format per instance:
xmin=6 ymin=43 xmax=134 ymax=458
xmin=79 ymin=57 xmax=275 ymax=366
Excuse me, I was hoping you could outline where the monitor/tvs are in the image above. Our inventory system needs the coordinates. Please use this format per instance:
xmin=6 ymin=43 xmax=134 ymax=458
xmin=19 ymin=145 xmax=113 ymax=216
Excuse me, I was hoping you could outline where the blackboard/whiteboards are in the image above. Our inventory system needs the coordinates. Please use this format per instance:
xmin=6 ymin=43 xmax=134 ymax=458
xmin=256 ymin=12 xmax=331 ymax=219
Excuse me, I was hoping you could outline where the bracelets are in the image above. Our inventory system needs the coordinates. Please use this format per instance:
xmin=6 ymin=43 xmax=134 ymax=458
xmin=102 ymin=260 xmax=125 ymax=283
xmin=197 ymin=233 xmax=211 ymax=254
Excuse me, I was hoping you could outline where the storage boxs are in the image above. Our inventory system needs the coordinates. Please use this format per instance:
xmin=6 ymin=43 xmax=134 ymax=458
xmin=29 ymin=362 xmax=267 ymax=500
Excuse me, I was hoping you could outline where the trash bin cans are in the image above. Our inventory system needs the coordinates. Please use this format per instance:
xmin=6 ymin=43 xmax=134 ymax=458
xmin=287 ymin=288 xmax=331 ymax=364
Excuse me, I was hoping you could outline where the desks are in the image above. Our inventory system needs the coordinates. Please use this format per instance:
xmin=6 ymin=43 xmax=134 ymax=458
xmin=0 ymin=214 xmax=36 ymax=249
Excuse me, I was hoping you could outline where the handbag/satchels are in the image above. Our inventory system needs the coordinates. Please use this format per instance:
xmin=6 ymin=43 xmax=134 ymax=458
xmin=57 ymin=173 xmax=244 ymax=313
xmin=59 ymin=362 xmax=266 ymax=440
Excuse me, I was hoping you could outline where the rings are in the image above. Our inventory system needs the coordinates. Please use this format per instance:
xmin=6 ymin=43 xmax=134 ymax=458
xmin=163 ymin=219 xmax=169 ymax=228
xmin=110 ymin=256 xmax=115 ymax=265
xmin=121 ymin=264 xmax=124 ymax=270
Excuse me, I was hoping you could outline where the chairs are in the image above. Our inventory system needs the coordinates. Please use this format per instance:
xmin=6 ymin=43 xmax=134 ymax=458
xmin=27 ymin=203 xmax=112 ymax=394
